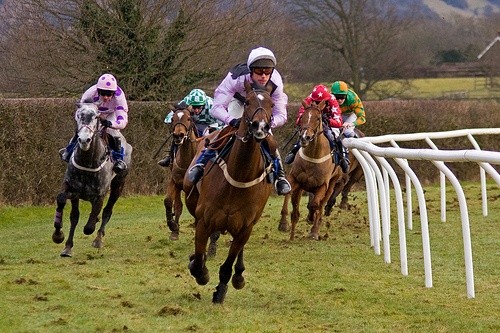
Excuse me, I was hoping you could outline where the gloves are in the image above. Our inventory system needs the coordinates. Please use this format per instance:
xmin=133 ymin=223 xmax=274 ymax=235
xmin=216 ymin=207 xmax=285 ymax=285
xmin=229 ymin=119 xmax=240 ymax=128
xmin=102 ymin=119 xmax=111 ymax=127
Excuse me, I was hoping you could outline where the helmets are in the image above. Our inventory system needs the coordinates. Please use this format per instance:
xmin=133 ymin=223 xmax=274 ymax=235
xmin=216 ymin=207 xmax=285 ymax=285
xmin=96 ymin=73 xmax=117 ymax=91
xmin=188 ymin=89 xmax=206 ymax=105
xmin=310 ymin=85 xmax=332 ymax=101
xmin=250 ymin=59 xmax=275 ymax=69
xmin=331 ymin=81 xmax=348 ymax=95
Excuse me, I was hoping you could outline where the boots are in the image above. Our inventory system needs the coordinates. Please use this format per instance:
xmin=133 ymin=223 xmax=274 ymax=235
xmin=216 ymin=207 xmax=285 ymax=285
xmin=271 ymin=148 xmax=289 ymax=194
xmin=285 ymin=146 xmax=299 ymax=163
xmin=63 ymin=135 xmax=79 ymax=161
xmin=189 ymin=148 xmax=213 ymax=182
xmin=337 ymin=141 xmax=350 ymax=173
xmin=158 ymin=141 xmax=177 ymax=167
xmin=109 ymin=136 xmax=125 ymax=172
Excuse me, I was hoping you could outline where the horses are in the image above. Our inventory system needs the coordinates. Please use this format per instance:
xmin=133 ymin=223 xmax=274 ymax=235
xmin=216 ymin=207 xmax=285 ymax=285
xmin=52 ymin=97 xmax=133 ymax=258
xmin=278 ymin=98 xmax=365 ymax=241
xmin=163 ymin=75 xmax=279 ymax=305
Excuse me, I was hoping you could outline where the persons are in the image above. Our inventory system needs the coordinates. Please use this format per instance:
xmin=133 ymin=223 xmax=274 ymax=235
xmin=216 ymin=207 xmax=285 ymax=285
xmin=61 ymin=73 xmax=129 ymax=172
xmin=188 ymin=46 xmax=290 ymax=195
xmin=329 ymin=81 xmax=367 ymax=139
xmin=284 ymin=85 xmax=350 ymax=173
xmin=159 ymin=89 xmax=224 ymax=166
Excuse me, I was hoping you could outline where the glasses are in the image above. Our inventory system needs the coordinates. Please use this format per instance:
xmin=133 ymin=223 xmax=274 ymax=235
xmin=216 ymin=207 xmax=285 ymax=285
xmin=252 ymin=68 xmax=272 ymax=75
xmin=191 ymin=105 xmax=203 ymax=109
xmin=334 ymin=94 xmax=346 ymax=100
xmin=99 ymin=90 xmax=115 ymax=97
